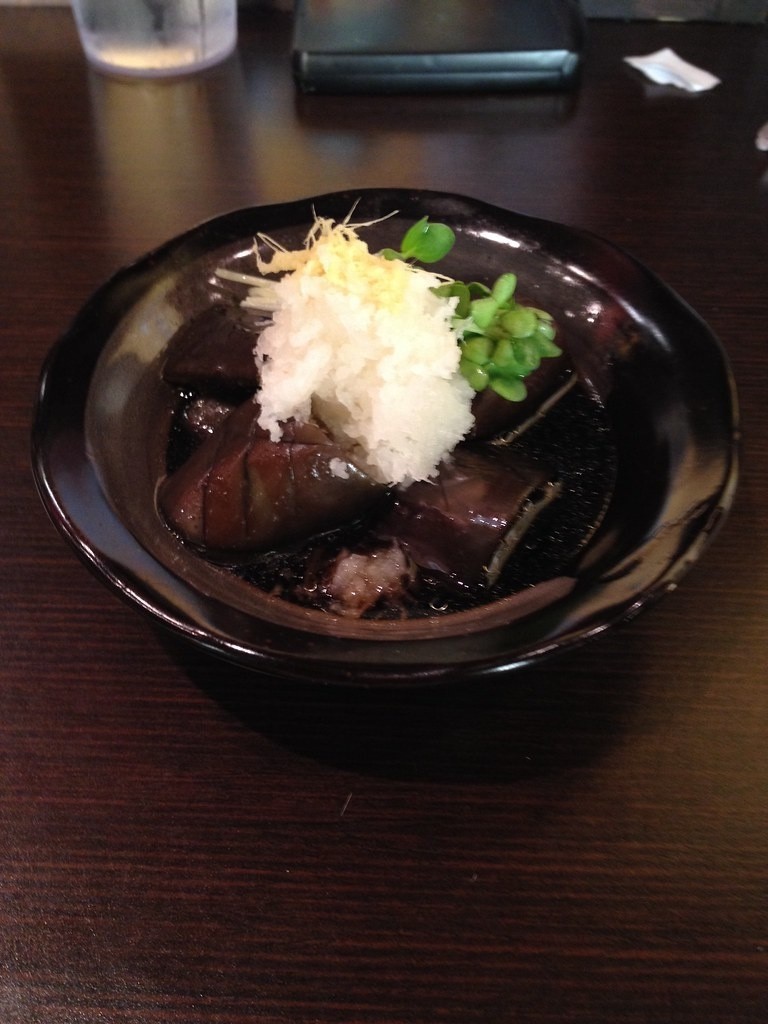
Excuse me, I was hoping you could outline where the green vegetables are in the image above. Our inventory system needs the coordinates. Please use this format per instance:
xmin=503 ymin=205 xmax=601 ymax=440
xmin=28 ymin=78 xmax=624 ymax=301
xmin=378 ymin=213 xmax=561 ymax=401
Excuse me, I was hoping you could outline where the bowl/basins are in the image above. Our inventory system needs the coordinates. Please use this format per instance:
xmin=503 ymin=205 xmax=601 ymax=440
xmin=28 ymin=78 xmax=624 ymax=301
xmin=34 ymin=181 xmax=747 ymax=741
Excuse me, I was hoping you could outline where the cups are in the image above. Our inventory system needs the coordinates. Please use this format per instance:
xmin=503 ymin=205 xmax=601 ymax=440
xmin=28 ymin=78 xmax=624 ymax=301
xmin=66 ymin=0 xmax=241 ymax=78
